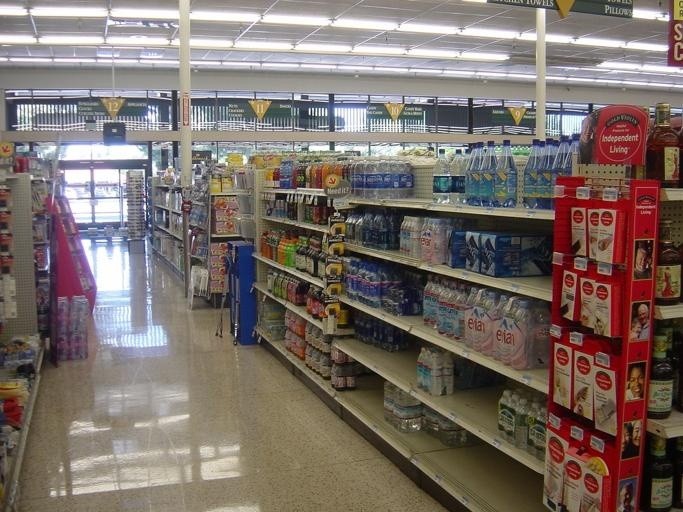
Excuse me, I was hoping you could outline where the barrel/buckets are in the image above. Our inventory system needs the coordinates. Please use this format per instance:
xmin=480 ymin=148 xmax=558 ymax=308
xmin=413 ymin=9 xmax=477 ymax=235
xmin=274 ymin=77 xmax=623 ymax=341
xmin=392 ymin=388 xmax=422 ymax=433
xmin=438 ymin=417 xmax=472 ymax=448
xmin=425 ymin=409 xmax=443 ymax=435
xmin=383 ymin=380 xmax=398 ymax=423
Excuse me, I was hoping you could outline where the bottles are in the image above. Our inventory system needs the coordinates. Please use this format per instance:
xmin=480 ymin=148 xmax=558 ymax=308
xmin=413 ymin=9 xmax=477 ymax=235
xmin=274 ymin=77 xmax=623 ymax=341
xmin=498 ymin=387 xmax=549 ymax=462
xmin=260 ymin=227 xmax=328 ymax=280
xmin=495 ymin=139 xmax=518 ymax=208
xmin=646 ymin=319 xmax=683 ymax=418
xmin=655 ymin=241 xmax=681 ymax=305
xmin=266 ymin=267 xmax=326 ymax=321
xmin=659 ymin=219 xmax=672 ymax=241
xmin=399 ymin=215 xmax=479 ymax=266
xmin=342 ymin=256 xmax=424 ymax=316
xmin=353 ymin=311 xmax=407 ymax=352
xmin=423 ymin=273 xmax=551 ymax=370
xmin=450 ymin=149 xmax=467 ymax=204
xmin=416 ymin=346 xmax=455 ymax=396
xmin=645 ymin=103 xmax=681 ymax=188
xmin=344 ymin=210 xmax=400 ymax=249
xmin=341 ymin=159 xmax=414 ymax=200
xmin=432 ymin=148 xmax=451 ymax=205
xmin=383 ymin=380 xmax=467 ymax=447
xmin=284 ymin=309 xmax=357 ymax=391
xmin=522 ymin=134 xmax=580 ymax=209
xmin=264 ymin=159 xmax=346 ymax=189
xmin=643 ymin=436 xmax=683 ymax=512
xmin=465 ymin=140 xmax=498 ymax=207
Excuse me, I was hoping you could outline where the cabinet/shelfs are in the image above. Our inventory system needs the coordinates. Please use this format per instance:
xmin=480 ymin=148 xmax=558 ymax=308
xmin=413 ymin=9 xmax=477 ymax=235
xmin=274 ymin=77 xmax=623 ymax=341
xmin=0 ymin=170 xmax=54 ymax=351
